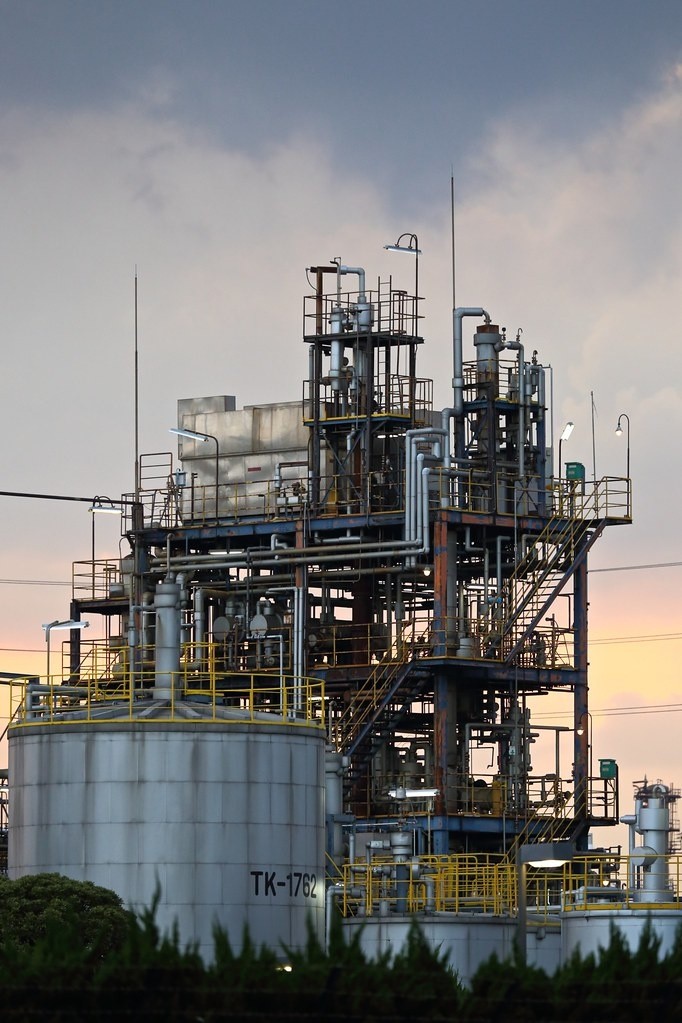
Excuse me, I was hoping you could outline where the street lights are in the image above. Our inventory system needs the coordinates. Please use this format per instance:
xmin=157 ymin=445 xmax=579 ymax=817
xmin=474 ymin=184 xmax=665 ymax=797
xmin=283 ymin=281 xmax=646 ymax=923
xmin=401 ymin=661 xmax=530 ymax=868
xmin=388 ymin=788 xmax=440 ymax=855
xmin=577 ymin=713 xmax=593 ymax=819
xmin=382 ymin=233 xmax=422 ymax=336
xmin=616 ymin=413 xmax=630 ymax=517
xmin=557 ymin=423 xmax=574 ymax=513
xmin=88 ymin=495 xmax=125 ymax=599
xmin=169 ymin=428 xmax=220 ymax=525
xmin=412 ymin=552 xmax=432 ymax=654
xmin=516 ymin=843 xmax=573 ymax=967
xmin=42 ymin=620 xmax=90 ymax=706
